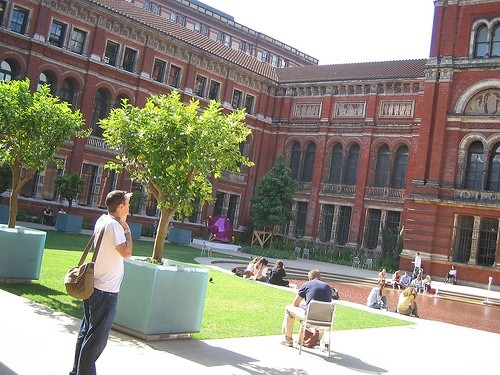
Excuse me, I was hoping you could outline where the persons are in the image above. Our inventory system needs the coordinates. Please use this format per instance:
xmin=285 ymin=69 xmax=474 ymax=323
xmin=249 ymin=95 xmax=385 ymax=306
xmin=446 ymin=265 xmax=456 ymax=282
xmin=43 ymin=204 xmax=52 ymax=225
xmin=413 ymin=275 xmax=423 ymax=293
xmin=394 ymin=287 xmax=419 ymax=317
xmin=58 ymin=207 xmax=66 ymax=214
xmin=167 ymin=222 xmax=174 ymax=238
xmin=268 ymin=260 xmax=289 ymax=287
xmin=254 ymin=257 xmax=270 ymax=282
xmin=412 ymin=252 xmax=422 ymax=278
xmin=401 ymin=271 xmax=412 ymax=288
xmin=154 ymin=219 xmax=159 ymax=236
xmin=423 ymin=274 xmax=433 ymax=293
xmin=69 ymin=190 xmax=133 ymax=375
xmin=391 ymin=272 xmax=401 ymax=290
xmin=367 ymin=282 xmax=386 ymax=309
xmin=244 ymin=258 xmax=260 ymax=280
xmin=279 ymin=269 xmax=331 ymax=348
xmin=378 ymin=269 xmax=387 ymax=287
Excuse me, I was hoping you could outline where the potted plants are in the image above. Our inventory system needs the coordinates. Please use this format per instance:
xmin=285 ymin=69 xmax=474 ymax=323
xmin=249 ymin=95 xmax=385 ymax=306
xmin=53 ymin=172 xmax=87 ymax=233
xmin=167 ymin=204 xmax=199 ymax=245
xmin=0 ymin=163 xmax=13 ymax=224
xmin=127 ymin=191 xmax=143 ymax=240
xmin=0 ymin=76 xmax=93 ymax=284
xmin=96 ymin=88 xmax=255 ymax=340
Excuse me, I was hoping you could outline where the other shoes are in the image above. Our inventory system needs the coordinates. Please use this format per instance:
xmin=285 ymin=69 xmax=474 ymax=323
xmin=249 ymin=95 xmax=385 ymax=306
xmin=281 ymin=339 xmax=293 ymax=346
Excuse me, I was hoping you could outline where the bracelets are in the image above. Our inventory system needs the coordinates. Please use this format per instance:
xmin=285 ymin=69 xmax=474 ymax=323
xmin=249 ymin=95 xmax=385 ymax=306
xmin=124 ymin=226 xmax=131 ymax=234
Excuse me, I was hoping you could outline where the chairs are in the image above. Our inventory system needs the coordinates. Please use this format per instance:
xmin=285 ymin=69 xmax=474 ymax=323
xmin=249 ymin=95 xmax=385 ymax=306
xmin=201 ymin=242 xmax=212 ymax=258
xmin=299 ymin=300 xmax=336 ymax=359
xmin=294 ymin=247 xmax=310 ymax=260
xmin=352 ymin=257 xmax=372 ymax=270
xmin=40 ymin=210 xmax=46 ymax=224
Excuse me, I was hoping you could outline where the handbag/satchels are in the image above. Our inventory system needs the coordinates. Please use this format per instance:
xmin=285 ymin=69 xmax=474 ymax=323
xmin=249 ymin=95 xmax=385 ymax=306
xmin=65 ymin=262 xmax=95 ymax=300
xmin=429 ymin=289 xmax=436 ymax=294
xmin=298 ymin=327 xmax=319 ymax=348
